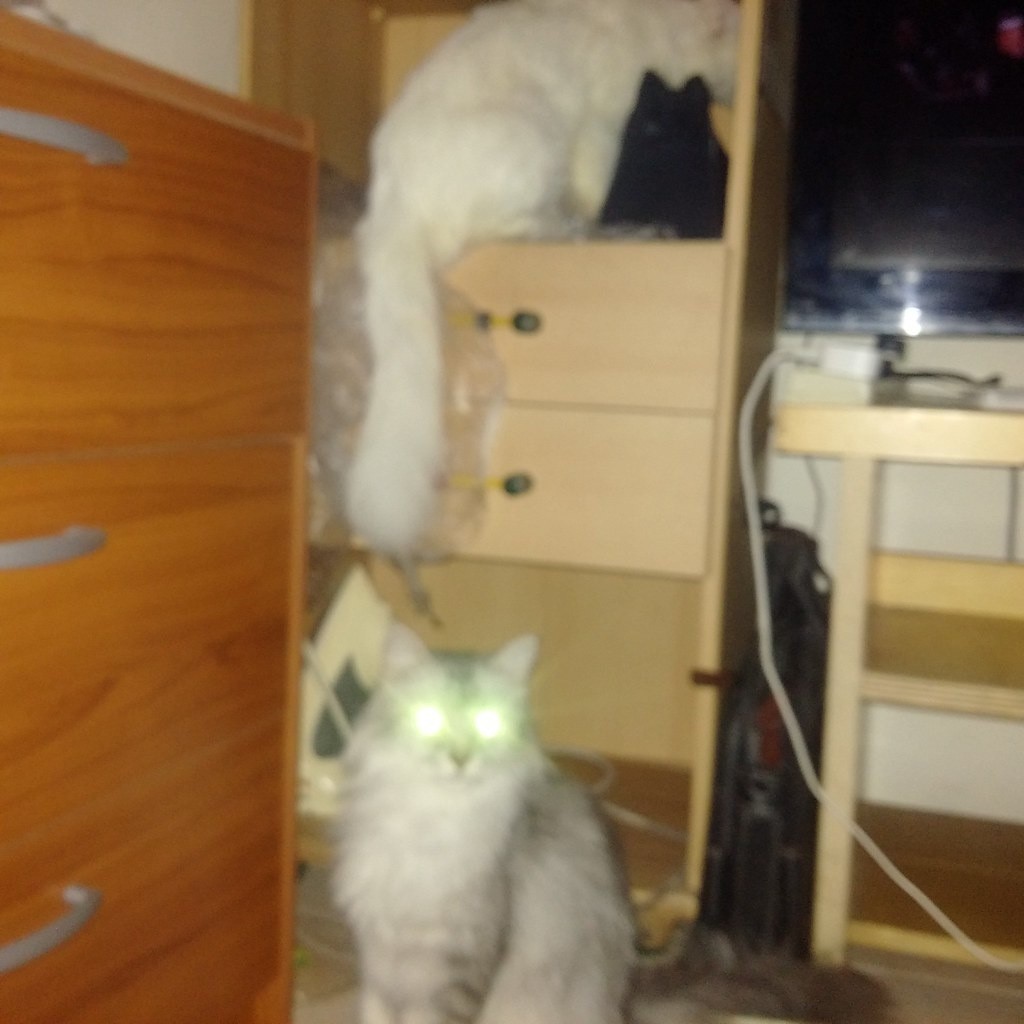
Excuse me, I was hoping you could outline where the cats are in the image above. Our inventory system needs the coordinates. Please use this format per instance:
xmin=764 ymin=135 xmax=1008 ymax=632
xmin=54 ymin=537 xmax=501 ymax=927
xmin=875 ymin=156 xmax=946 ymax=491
xmin=341 ymin=0 xmax=740 ymax=553
xmin=329 ymin=621 xmax=633 ymax=1024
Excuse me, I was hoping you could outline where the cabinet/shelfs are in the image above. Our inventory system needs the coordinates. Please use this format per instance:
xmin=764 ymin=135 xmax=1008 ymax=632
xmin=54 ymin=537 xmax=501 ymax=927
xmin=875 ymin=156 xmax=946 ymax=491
xmin=0 ymin=0 xmax=320 ymax=1024
xmin=240 ymin=0 xmax=796 ymax=951
xmin=768 ymin=372 xmax=1024 ymax=975
